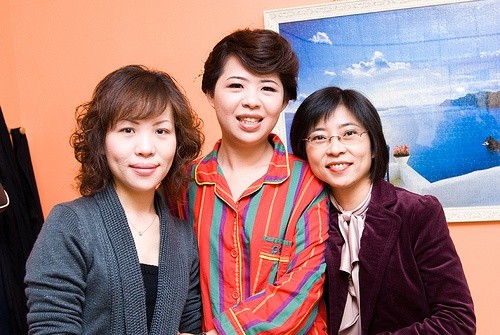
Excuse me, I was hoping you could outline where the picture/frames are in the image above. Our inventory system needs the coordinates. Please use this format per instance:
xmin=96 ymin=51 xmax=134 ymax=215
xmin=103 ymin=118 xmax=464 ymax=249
xmin=262 ymin=0 xmax=500 ymax=225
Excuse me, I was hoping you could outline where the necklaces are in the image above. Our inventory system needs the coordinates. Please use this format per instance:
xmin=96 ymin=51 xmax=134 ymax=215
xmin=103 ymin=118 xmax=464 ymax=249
xmin=125 ymin=215 xmax=158 ymax=237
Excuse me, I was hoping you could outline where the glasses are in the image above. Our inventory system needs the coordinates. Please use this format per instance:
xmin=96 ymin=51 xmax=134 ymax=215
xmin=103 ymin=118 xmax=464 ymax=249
xmin=304 ymin=129 xmax=368 ymax=145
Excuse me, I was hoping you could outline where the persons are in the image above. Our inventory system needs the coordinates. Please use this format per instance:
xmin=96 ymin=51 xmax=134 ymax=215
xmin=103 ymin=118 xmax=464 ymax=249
xmin=169 ymin=28 xmax=329 ymax=335
xmin=289 ymin=86 xmax=476 ymax=335
xmin=23 ymin=64 xmax=204 ymax=335
